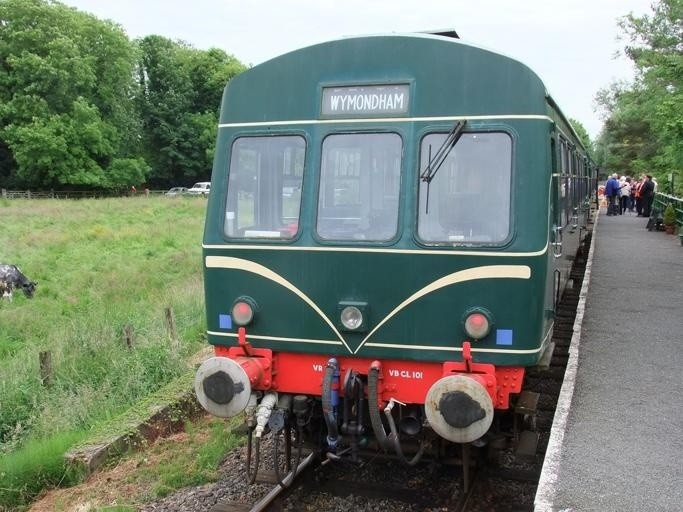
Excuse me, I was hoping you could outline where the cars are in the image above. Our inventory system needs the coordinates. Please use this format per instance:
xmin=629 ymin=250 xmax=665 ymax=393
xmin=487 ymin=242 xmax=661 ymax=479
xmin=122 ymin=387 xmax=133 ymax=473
xmin=281 ymin=174 xmax=303 ymax=197
xmin=164 ymin=188 xmax=187 ymax=197
xmin=188 ymin=182 xmax=211 ymax=196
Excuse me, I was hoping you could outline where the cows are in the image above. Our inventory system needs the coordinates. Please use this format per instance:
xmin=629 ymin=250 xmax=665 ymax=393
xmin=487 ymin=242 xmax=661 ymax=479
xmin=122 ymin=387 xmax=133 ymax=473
xmin=0 ymin=264 xmax=39 ymax=302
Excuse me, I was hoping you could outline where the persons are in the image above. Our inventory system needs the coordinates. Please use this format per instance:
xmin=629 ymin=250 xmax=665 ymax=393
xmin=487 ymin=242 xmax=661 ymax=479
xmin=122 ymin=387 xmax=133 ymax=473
xmin=130 ymin=186 xmax=136 ymax=196
xmin=605 ymin=172 xmax=658 ymax=217
xmin=144 ymin=186 xmax=149 ymax=198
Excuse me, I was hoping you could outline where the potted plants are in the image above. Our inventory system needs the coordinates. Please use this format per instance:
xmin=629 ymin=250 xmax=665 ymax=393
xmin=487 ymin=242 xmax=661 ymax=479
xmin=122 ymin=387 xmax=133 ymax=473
xmin=662 ymin=203 xmax=677 ymax=234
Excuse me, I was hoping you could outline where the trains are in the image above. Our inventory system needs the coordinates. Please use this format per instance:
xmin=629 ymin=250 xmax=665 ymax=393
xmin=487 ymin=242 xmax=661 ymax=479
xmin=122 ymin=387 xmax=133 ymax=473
xmin=194 ymin=31 xmax=599 ymax=478
